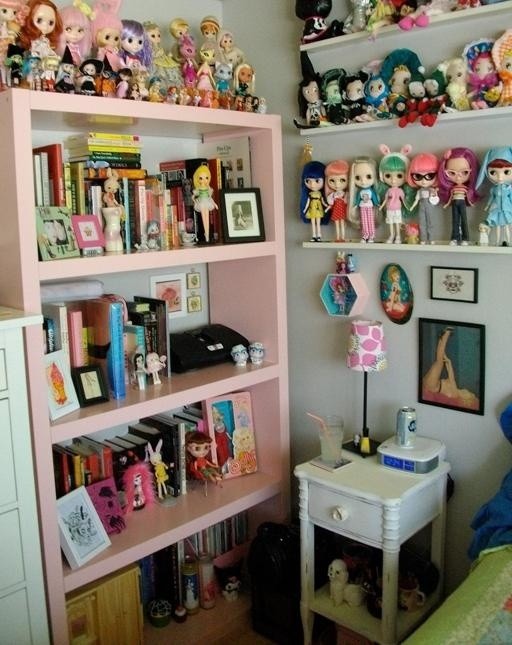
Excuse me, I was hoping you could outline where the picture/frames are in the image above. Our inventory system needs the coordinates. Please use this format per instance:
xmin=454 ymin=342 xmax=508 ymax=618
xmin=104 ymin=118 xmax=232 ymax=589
xmin=219 ymin=187 xmax=266 ymax=243
xmin=35 ymin=206 xmax=80 ymax=260
xmin=186 ymin=272 xmax=201 ymax=288
xmin=150 ymin=273 xmax=187 ymax=319
xmin=56 ymin=485 xmax=112 ymax=570
xmin=72 ymin=365 xmax=110 ymax=408
xmin=71 ymin=214 xmax=107 ymax=249
xmin=379 ymin=264 xmax=414 ymax=327
xmin=46 ymin=349 xmax=80 ymax=422
xmin=188 ymin=295 xmax=201 ymax=312
xmin=417 ymin=317 xmax=486 ymax=416
xmin=430 ymin=265 xmax=478 ymax=304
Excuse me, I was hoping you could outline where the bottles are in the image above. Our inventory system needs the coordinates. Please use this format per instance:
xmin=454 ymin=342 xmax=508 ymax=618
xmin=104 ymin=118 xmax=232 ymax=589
xmin=182 ymin=554 xmax=216 ymax=616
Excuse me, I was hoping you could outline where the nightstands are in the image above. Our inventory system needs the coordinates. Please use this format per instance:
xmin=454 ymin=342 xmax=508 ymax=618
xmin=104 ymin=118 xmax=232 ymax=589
xmin=294 ymin=439 xmax=451 ymax=645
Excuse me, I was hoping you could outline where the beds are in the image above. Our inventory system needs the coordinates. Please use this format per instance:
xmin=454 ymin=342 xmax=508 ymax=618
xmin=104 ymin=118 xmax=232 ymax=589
xmin=400 ymin=406 xmax=512 ymax=645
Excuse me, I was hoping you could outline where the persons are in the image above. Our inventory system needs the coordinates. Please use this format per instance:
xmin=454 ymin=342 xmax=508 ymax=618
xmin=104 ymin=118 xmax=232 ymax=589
xmin=421 ymin=326 xmax=480 ymax=410
xmin=383 ymin=266 xmax=404 ymax=314
xmin=296 ymin=0 xmax=512 ymax=245
xmin=0 ymin=1 xmax=266 ymax=112
xmin=299 ymin=144 xmax=512 ymax=246
xmin=184 ymin=430 xmax=224 ymax=488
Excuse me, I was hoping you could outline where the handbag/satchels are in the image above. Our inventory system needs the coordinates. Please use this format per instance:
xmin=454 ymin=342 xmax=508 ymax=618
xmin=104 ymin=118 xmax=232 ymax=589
xmin=251 ymin=521 xmax=336 ymax=645
xmin=170 ymin=324 xmax=250 ymax=374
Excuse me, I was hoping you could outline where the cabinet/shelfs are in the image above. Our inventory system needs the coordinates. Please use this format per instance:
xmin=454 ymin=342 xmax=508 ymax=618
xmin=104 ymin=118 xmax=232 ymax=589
xmin=0 ymin=87 xmax=294 ymax=645
xmin=299 ymin=0 xmax=512 ymax=253
xmin=0 ymin=305 xmax=51 ymax=645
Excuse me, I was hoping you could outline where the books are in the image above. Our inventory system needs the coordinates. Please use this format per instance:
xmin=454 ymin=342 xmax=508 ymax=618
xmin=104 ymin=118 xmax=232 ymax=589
xmin=165 ymin=511 xmax=247 ymax=609
xmin=39 ymin=294 xmax=171 ymax=397
xmin=52 ymin=391 xmax=204 ymax=570
xmin=32 ymin=132 xmax=224 ymax=251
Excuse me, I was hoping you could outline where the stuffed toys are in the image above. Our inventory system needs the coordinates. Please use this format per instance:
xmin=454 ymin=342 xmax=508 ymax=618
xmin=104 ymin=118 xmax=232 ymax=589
xmin=297 ymin=28 xmax=512 ymax=127
xmin=299 ymin=0 xmax=484 ymax=44
xmin=326 ymin=558 xmax=360 ymax=607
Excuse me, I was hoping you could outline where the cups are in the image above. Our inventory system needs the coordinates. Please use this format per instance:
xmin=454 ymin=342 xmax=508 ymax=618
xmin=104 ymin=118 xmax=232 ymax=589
xmin=399 ymin=584 xmax=426 ymax=612
xmin=319 ymin=416 xmax=345 ymax=463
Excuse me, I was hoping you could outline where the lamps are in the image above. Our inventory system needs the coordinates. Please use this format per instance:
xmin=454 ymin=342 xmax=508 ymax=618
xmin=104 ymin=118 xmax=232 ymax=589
xmin=341 ymin=319 xmax=388 ymax=458
